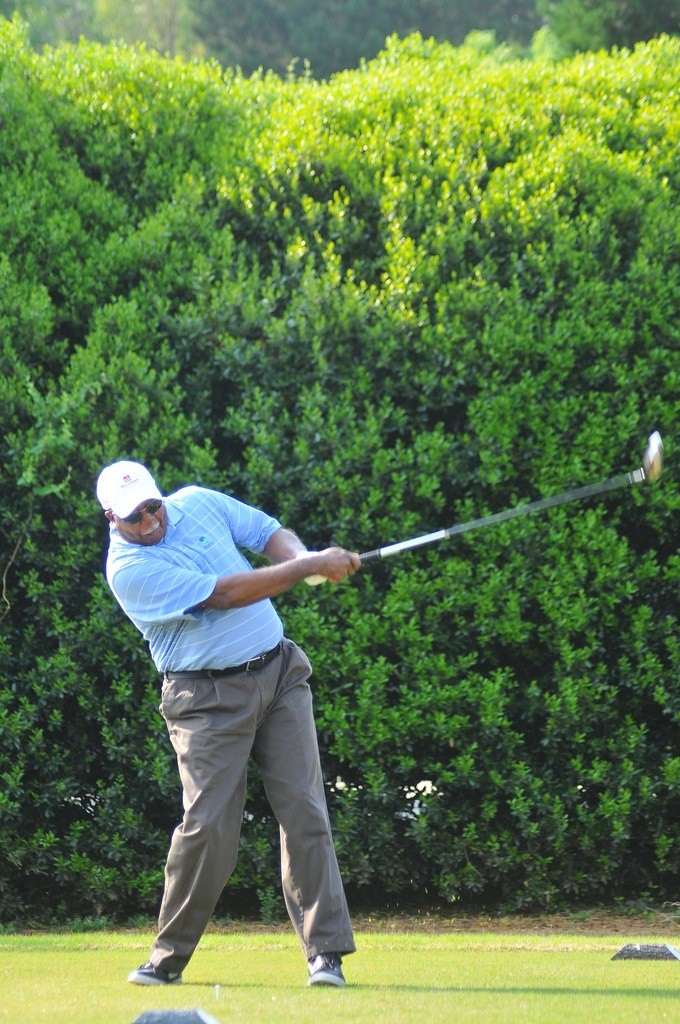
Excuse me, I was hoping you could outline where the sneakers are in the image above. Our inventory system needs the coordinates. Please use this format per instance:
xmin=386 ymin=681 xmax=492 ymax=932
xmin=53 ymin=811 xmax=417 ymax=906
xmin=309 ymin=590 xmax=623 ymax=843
xmin=128 ymin=961 xmax=182 ymax=985
xmin=308 ymin=951 xmax=345 ymax=985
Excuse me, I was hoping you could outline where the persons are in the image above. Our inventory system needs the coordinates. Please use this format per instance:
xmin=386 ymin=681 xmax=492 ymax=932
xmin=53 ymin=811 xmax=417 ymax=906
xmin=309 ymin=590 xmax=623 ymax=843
xmin=96 ymin=459 xmax=362 ymax=990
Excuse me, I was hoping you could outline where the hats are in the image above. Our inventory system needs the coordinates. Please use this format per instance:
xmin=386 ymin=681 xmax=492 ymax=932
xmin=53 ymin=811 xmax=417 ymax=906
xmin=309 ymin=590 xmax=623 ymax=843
xmin=97 ymin=460 xmax=163 ymax=519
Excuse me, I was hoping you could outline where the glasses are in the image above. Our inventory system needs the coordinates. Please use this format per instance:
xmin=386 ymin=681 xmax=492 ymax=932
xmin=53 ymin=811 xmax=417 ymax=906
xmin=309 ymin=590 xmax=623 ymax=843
xmin=111 ymin=499 xmax=162 ymax=525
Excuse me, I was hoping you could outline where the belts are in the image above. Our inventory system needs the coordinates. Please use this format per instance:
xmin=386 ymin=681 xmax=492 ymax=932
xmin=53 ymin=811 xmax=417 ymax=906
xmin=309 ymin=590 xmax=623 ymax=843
xmin=167 ymin=644 xmax=280 ymax=678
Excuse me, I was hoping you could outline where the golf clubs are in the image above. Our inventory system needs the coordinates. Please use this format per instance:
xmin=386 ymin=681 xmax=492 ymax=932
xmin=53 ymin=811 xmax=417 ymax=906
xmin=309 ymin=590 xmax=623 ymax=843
xmin=305 ymin=430 xmax=665 ymax=589
